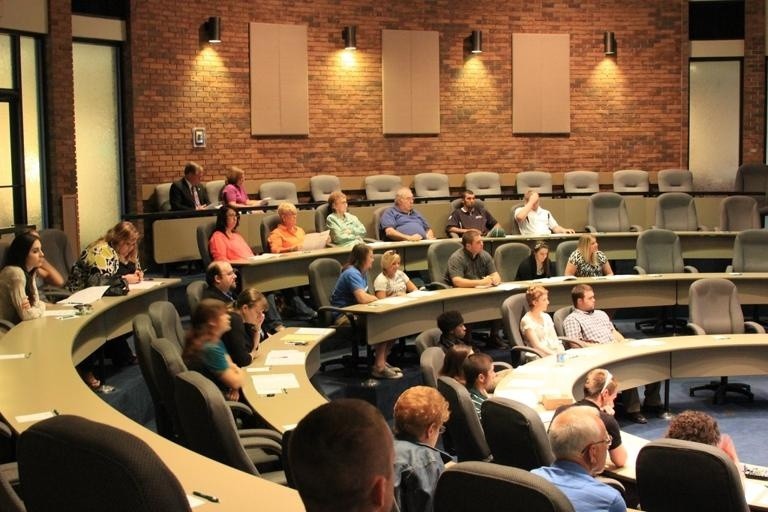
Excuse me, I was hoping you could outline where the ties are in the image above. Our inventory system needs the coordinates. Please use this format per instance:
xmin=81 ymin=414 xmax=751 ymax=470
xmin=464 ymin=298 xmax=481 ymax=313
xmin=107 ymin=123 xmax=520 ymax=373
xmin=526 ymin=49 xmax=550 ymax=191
xmin=192 ymin=186 xmax=201 ymax=208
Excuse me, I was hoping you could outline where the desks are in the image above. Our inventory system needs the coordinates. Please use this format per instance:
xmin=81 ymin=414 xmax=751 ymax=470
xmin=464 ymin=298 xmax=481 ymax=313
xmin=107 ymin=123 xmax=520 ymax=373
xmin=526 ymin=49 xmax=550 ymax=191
xmin=494 ymin=333 xmax=767 ymax=511
xmin=1 ymin=278 xmax=306 ymax=512
xmin=240 ymin=325 xmax=336 ymax=435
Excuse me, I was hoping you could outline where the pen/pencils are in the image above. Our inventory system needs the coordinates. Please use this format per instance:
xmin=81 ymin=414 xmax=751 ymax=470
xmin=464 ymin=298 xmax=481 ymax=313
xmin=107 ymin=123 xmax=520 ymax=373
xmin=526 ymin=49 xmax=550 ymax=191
xmin=142 ymin=266 xmax=151 ymax=273
xmin=68 ymin=302 xmax=83 ymax=304
xmin=368 ymin=305 xmax=378 ymax=306
xmin=26 ymin=352 xmax=32 ymax=358
xmin=295 ymin=342 xmax=309 ymax=345
xmin=283 ymin=388 xmax=287 ymax=394
xmin=193 ymin=491 xmax=219 ymax=503
xmin=54 ymin=409 xmax=60 ymax=416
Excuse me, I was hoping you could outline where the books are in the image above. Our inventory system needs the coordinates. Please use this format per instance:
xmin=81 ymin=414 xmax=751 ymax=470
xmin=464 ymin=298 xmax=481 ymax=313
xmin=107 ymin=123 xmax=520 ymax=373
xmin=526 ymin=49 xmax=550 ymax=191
xmin=255 ymin=197 xmax=271 ymax=206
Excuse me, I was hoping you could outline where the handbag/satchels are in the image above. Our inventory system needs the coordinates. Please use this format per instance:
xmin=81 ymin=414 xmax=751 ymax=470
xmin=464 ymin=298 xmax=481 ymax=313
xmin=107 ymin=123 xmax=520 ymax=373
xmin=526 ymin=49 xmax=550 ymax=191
xmin=84 ymin=273 xmax=130 ymax=296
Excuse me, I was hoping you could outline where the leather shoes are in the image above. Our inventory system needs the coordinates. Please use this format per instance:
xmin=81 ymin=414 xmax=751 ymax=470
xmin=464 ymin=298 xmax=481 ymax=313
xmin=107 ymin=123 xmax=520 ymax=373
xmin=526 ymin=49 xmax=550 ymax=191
xmin=643 ymin=405 xmax=670 ymax=418
xmin=626 ymin=412 xmax=647 ymax=424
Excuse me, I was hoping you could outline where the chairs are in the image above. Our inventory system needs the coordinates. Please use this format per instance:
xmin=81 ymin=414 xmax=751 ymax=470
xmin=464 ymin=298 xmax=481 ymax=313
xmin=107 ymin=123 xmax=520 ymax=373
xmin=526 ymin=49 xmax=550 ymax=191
xmin=438 ymin=373 xmax=491 ymax=460
xmin=501 ymin=293 xmax=546 ymax=364
xmin=553 ymin=304 xmax=586 ymax=349
xmin=432 ymin=460 xmax=574 ymax=510
xmin=415 ymin=328 xmax=513 ymax=371
xmin=37 ymin=228 xmax=76 ymax=291
xmin=186 ymin=280 xmax=208 ymax=318
xmin=309 ymin=259 xmax=378 ymax=383
xmin=420 ymin=346 xmax=447 ymax=390
xmin=132 ymin=313 xmax=156 ymax=388
xmin=635 ymin=437 xmax=750 ymax=512
xmin=171 ymin=370 xmax=286 ymax=486
xmin=149 ymin=301 xmax=185 ymax=355
xmin=14 ymin=416 xmax=190 ymax=512
xmin=150 ymin=336 xmax=283 ymax=437
xmin=686 ymin=279 xmax=766 ymax=406
xmin=481 ymin=398 xmax=625 ymax=494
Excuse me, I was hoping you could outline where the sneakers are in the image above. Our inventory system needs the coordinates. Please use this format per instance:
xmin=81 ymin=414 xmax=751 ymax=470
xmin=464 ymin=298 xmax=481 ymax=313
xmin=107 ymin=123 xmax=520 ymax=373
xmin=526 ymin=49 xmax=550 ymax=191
xmin=372 ymin=367 xmax=403 ymax=379
xmin=372 ymin=362 xmax=402 ymax=372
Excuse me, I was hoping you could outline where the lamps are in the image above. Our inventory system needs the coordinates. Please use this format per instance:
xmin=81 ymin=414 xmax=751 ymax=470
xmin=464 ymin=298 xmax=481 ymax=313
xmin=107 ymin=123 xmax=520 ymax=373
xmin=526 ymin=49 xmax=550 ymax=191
xmin=345 ymin=25 xmax=357 ymax=51
xmin=605 ymin=32 xmax=615 ymax=56
xmin=207 ymin=15 xmax=223 ymax=44
xmin=471 ymin=31 xmax=484 ymax=53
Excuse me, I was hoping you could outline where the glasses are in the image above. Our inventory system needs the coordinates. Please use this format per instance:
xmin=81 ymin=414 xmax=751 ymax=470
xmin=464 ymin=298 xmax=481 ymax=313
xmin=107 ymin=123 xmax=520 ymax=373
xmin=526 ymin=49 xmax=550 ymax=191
xmin=438 ymin=424 xmax=446 ymax=434
xmin=601 ymin=369 xmax=613 ymax=395
xmin=581 ymin=434 xmax=613 ymax=454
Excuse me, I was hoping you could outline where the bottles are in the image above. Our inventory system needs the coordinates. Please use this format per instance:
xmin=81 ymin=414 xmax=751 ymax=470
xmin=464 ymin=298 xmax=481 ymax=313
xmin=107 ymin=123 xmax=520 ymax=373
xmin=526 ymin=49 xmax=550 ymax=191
xmin=557 ymin=340 xmax=566 ymax=363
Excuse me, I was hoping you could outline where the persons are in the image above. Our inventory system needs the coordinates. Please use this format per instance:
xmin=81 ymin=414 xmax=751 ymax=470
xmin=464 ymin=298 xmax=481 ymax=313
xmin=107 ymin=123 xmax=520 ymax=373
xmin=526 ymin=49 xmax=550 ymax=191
xmin=438 ymin=230 xmax=507 ymax=431
xmin=208 ymin=205 xmax=319 ymax=333
xmin=1 ymin=220 xmax=145 ymax=327
xmin=515 ymin=190 xmax=576 ymax=235
xmin=536 ymin=366 xmax=749 ymax=512
xmin=325 ymin=191 xmax=366 ymax=246
xmin=267 ymin=200 xmax=332 ymax=254
xmin=182 ymin=260 xmax=267 ymax=424
xmin=379 ymin=187 xmax=437 ymax=283
xmin=330 ymin=242 xmax=419 ymax=379
xmin=446 ymin=189 xmax=500 ymax=238
xmin=206 ymin=204 xmax=223 ymax=210
xmin=286 ymin=384 xmax=453 ymax=511
xmin=169 ymin=162 xmax=213 ymax=211
xmin=513 ymin=234 xmax=664 ymax=424
xmin=218 ymin=168 xmax=268 ymax=208
